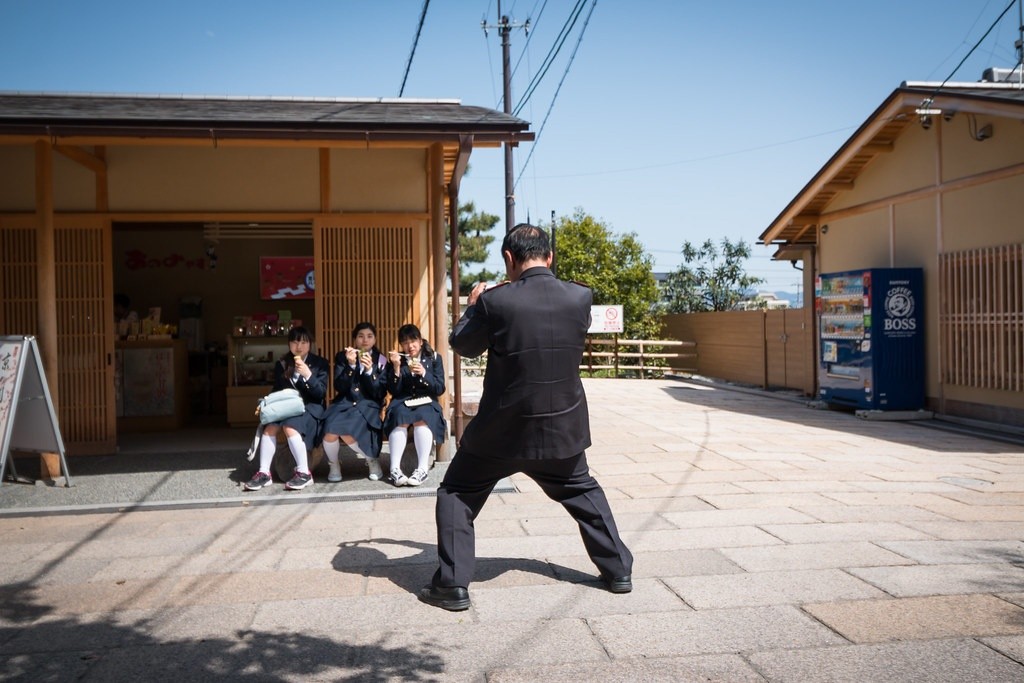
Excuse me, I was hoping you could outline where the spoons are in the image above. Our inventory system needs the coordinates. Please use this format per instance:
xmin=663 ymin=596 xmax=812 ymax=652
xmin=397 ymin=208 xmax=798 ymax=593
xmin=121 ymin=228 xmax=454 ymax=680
xmin=389 ymin=352 xmax=410 ymax=356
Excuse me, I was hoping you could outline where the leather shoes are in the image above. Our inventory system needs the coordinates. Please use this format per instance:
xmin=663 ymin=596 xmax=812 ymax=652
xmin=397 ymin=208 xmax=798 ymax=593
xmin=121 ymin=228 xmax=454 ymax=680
xmin=599 ymin=574 xmax=632 ymax=593
xmin=419 ymin=585 xmax=470 ymax=611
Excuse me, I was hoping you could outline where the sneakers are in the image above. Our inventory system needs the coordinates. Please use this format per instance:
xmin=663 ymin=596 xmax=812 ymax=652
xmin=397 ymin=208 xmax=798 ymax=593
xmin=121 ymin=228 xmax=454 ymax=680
xmin=390 ymin=468 xmax=408 ymax=486
xmin=407 ymin=469 xmax=429 ymax=485
xmin=244 ymin=472 xmax=273 ymax=491
xmin=366 ymin=456 xmax=383 ymax=481
xmin=285 ymin=471 xmax=314 ymax=490
xmin=328 ymin=459 xmax=342 ymax=482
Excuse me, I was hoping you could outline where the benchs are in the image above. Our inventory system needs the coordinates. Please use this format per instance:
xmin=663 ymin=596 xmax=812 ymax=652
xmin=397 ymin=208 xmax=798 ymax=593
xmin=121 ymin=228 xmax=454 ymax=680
xmin=276 ymin=423 xmax=415 ymax=482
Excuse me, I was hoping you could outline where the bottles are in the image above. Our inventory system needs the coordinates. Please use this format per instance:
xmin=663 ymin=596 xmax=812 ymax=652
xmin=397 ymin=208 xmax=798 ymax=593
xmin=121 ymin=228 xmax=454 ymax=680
xmin=822 ymin=279 xmax=863 ymax=335
xmin=241 ymin=320 xmax=296 ymax=336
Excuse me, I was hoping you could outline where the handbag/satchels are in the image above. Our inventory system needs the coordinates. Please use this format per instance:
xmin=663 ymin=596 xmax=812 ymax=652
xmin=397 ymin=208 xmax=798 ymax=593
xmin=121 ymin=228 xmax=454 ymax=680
xmin=259 ymin=389 xmax=306 ymax=425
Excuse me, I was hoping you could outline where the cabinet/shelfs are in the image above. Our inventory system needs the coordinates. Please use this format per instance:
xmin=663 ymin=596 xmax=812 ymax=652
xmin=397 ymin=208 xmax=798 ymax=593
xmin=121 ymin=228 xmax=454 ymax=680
xmin=226 ymin=334 xmax=298 ymax=430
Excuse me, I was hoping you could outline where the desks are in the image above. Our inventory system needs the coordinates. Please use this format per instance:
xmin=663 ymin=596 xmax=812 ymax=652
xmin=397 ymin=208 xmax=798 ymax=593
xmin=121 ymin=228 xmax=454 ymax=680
xmin=118 ymin=339 xmax=188 ymax=431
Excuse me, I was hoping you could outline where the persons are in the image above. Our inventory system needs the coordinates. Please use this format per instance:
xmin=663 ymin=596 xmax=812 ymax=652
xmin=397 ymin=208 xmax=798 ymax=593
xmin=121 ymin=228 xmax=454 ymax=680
xmin=418 ymin=222 xmax=635 ymax=612
xmin=322 ymin=320 xmax=392 ymax=483
xmin=386 ymin=324 xmax=447 ymax=488
xmin=243 ymin=327 xmax=332 ymax=490
